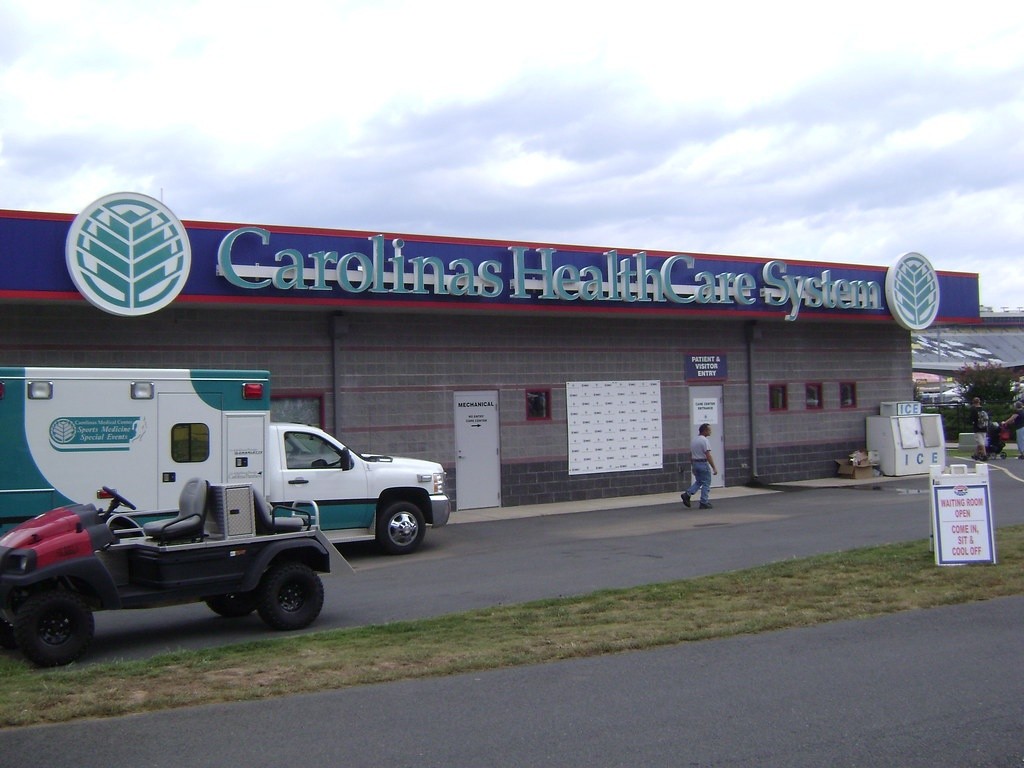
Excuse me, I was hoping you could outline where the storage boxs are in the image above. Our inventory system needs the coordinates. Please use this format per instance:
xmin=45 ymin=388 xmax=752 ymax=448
xmin=833 ymin=453 xmax=880 ymax=479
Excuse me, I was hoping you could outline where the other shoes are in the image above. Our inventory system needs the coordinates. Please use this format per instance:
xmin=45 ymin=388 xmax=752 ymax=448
xmin=698 ymin=502 xmax=713 ymax=509
xmin=971 ymin=454 xmax=978 ymax=460
xmin=681 ymin=492 xmax=691 ymax=508
xmin=1018 ymin=455 xmax=1024 ymax=460
xmin=979 ymin=455 xmax=987 ymax=461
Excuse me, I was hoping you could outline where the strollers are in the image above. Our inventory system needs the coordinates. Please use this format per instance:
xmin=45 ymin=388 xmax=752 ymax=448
xmin=977 ymin=422 xmax=1007 ymax=460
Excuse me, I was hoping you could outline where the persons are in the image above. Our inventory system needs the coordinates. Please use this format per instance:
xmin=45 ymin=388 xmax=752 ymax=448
xmin=1001 ymin=401 xmax=1024 ymax=460
xmin=681 ymin=424 xmax=717 ymax=509
xmin=968 ymin=397 xmax=989 ymax=461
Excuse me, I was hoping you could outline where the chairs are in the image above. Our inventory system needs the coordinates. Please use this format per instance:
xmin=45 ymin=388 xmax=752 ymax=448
xmin=140 ymin=476 xmax=210 ymax=540
xmin=251 ymin=473 xmax=313 ymax=528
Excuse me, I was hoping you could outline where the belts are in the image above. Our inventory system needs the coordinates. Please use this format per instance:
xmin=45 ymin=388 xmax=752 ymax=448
xmin=691 ymin=458 xmax=707 ymax=462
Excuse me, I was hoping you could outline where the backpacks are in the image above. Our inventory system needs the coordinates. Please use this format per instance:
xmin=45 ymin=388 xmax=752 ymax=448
xmin=976 ymin=411 xmax=989 ymax=429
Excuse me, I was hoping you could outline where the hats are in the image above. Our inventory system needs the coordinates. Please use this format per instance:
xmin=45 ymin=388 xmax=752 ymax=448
xmin=972 ymin=397 xmax=980 ymax=402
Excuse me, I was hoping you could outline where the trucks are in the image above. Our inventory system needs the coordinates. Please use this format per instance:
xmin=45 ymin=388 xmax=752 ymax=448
xmin=1 ymin=366 xmax=454 ymax=557
